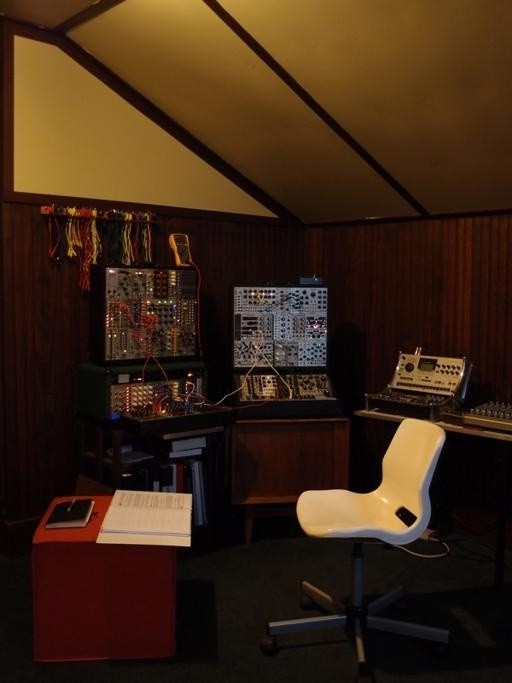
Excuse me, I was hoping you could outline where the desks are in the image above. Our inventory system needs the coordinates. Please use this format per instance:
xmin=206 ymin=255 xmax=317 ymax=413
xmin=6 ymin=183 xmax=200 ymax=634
xmin=352 ymin=402 xmax=512 ymax=548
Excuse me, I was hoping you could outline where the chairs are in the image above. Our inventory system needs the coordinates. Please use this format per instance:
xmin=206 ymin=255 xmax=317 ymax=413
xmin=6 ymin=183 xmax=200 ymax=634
xmin=261 ymin=419 xmax=454 ymax=676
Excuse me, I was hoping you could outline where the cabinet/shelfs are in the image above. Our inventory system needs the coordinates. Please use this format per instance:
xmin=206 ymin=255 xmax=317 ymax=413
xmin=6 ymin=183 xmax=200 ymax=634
xmin=80 ymin=363 xmax=238 ymax=551
xmin=234 ymin=422 xmax=351 ymax=543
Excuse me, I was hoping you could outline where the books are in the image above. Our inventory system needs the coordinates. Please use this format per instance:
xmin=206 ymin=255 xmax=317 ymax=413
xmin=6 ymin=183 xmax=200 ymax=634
xmin=95 ymin=489 xmax=192 ymax=547
xmin=44 ymin=498 xmax=94 ymax=528
xmin=110 ymin=436 xmax=205 ymax=525
